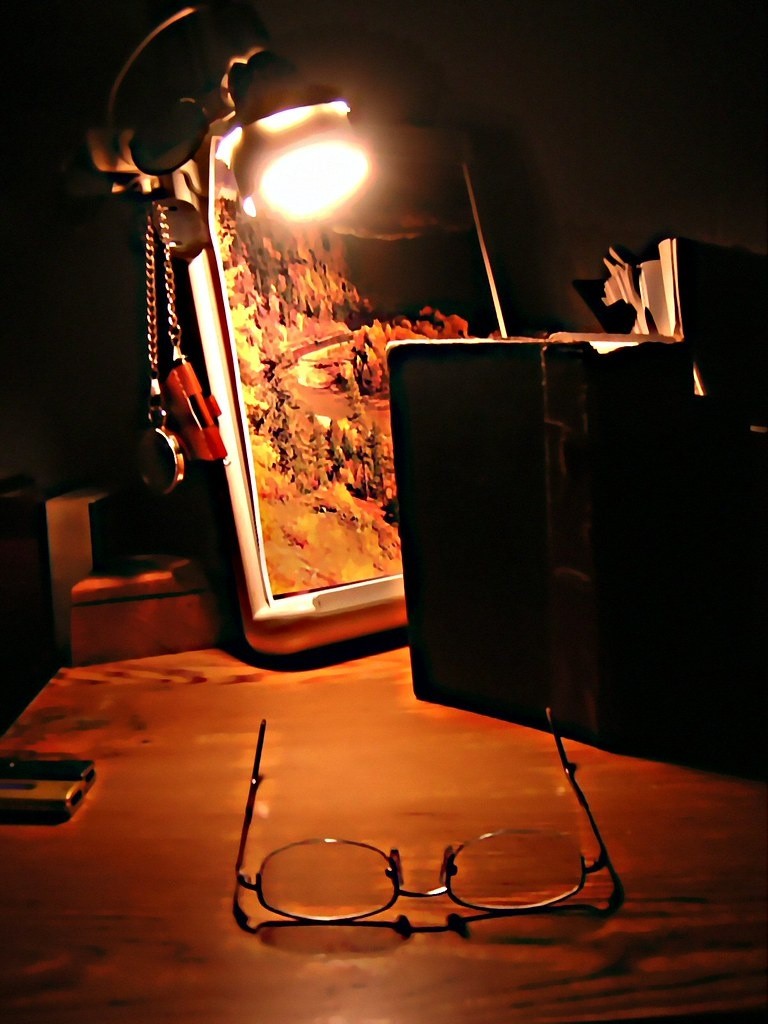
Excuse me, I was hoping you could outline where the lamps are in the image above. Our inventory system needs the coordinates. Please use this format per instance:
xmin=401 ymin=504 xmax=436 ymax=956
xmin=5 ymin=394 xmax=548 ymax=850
xmin=127 ymin=47 xmax=374 ymax=225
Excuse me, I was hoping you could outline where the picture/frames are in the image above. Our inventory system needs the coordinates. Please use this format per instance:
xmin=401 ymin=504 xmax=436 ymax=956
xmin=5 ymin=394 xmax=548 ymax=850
xmin=185 ymin=106 xmax=530 ymax=655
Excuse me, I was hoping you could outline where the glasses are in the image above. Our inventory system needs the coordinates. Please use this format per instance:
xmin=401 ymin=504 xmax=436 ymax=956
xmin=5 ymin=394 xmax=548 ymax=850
xmin=232 ymin=706 xmax=625 ymax=933
